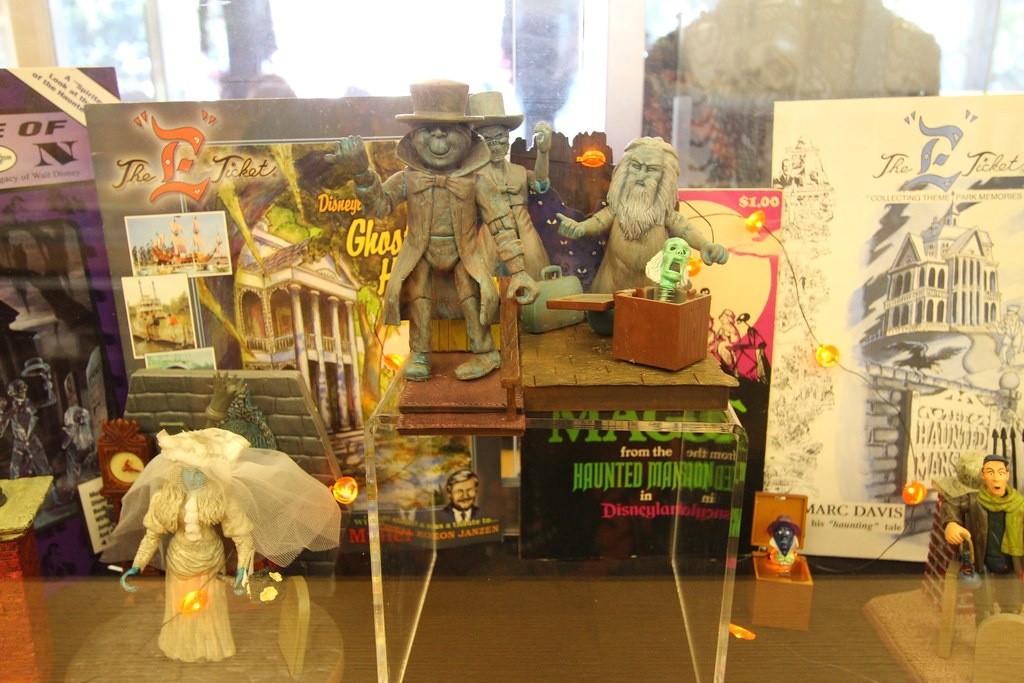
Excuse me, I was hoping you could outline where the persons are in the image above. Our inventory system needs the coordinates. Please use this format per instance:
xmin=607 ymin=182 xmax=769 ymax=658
xmin=465 ymin=92 xmax=553 ymax=284
xmin=942 ymin=455 xmax=1024 ymax=630
xmin=118 ymin=439 xmax=258 ymax=663
xmin=556 ymin=135 xmax=729 ymax=338
xmin=341 ymin=83 xmax=538 ymax=381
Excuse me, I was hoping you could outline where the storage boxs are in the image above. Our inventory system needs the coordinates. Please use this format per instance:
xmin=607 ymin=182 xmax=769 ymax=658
xmin=543 ymin=286 xmax=711 ymax=372
xmin=750 ymin=492 xmax=815 ymax=631
